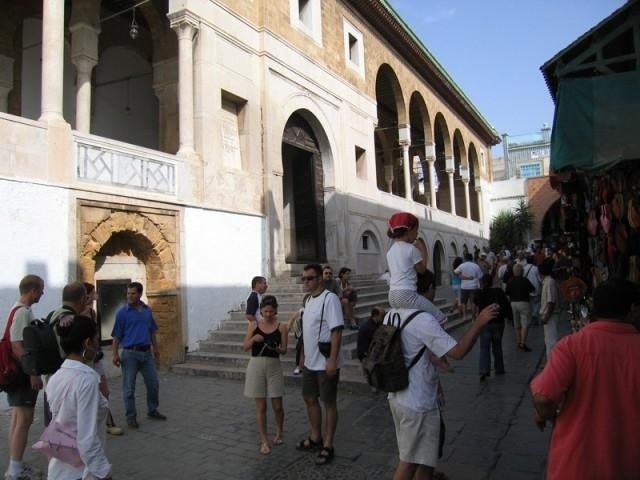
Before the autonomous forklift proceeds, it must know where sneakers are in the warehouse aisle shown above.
[294,367,301,374]
[23,464,44,474]
[4,472,42,480]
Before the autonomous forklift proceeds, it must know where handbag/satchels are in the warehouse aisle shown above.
[32,420,90,467]
[316,340,332,357]
[0,341,26,387]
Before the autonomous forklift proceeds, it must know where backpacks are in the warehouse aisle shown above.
[363,309,429,392]
[22,310,76,375]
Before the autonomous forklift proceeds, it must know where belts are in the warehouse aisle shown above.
[122,344,150,351]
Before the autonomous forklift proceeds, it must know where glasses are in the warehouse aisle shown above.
[301,276,320,281]
[325,272,332,275]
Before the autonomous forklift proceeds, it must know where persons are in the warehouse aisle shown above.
[381,212,462,374]
[245,276,268,324]
[112,278,168,427]
[384,267,503,480]
[43,280,87,436]
[292,293,312,374]
[241,295,288,454]
[1,273,48,480]
[293,263,345,463]
[79,283,124,436]
[381,209,462,374]
[322,265,361,330]
[358,305,385,391]
[44,312,111,480]
[450,240,590,382]
[528,276,639,480]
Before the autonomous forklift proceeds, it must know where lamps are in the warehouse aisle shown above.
[129,8,139,40]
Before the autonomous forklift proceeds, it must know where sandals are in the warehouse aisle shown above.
[260,441,271,455]
[273,435,283,444]
[315,445,334,466]
[295,437,321,450]
[519,343,532,352]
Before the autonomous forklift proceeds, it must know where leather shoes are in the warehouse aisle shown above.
[105,425,123,435]
[127,416,138,427]
[147,409,166,420]
